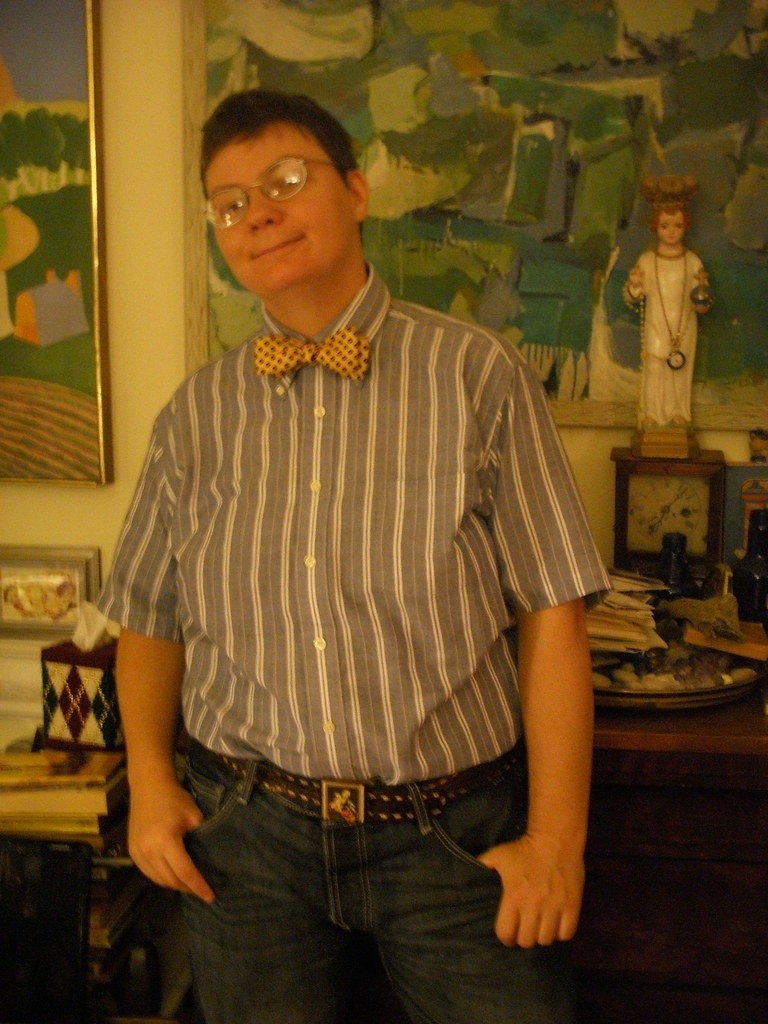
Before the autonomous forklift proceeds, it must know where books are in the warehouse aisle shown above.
[1,737,133,867]
[587,571,672,653]
[683,621,768,660]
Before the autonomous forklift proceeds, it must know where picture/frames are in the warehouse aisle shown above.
[0,0,112,485]
[0,546,99,643]
[180,0,768,429]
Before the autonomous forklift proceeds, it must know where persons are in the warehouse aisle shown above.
[622,175,716,429]
[97,90,614,1024]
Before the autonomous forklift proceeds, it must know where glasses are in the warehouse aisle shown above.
[203,155,335,229]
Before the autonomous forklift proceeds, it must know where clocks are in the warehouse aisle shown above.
[611,446,727,575]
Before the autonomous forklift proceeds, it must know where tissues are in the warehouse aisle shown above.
[40,600,126,752]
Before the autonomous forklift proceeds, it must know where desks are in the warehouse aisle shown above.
[534,706,768,1024]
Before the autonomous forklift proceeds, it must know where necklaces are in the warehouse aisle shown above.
[655,242,688,370]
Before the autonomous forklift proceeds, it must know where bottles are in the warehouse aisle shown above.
[652,532,701,603]
[733,509,768,627]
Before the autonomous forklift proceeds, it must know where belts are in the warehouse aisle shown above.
[195,750,517,825]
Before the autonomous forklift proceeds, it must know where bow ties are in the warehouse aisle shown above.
[254,327,374,384]
